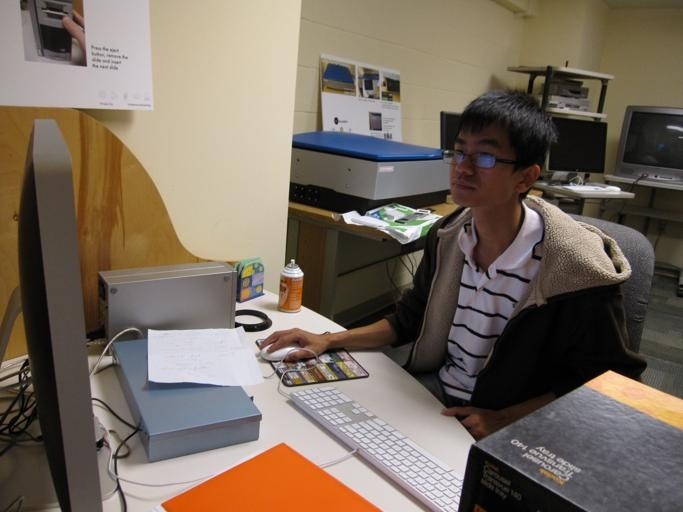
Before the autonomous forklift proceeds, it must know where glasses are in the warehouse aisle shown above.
[439,148,521,170]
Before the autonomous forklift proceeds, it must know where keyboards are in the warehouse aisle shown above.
[289,385,462,512]
[562,185,607,192]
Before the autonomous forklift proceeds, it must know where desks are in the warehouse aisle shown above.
[287,184,542,327]
[2,100,475,512]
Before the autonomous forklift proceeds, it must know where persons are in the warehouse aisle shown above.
[261,88,648,439]
[62,8,86,66]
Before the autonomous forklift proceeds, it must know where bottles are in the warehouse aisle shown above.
[276,255,307,313]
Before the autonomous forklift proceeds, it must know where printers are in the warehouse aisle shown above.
[288,130,451,216]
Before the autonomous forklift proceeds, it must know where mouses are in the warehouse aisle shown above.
[259,341,301,362]
[606,185,621,192]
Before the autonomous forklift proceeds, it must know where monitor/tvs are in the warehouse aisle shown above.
[545,117,608,174]
[616,104,683,186]
[0,119,105,512]
[440,110,463,150]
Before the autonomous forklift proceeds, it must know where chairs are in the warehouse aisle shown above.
[556,209,656,366]
